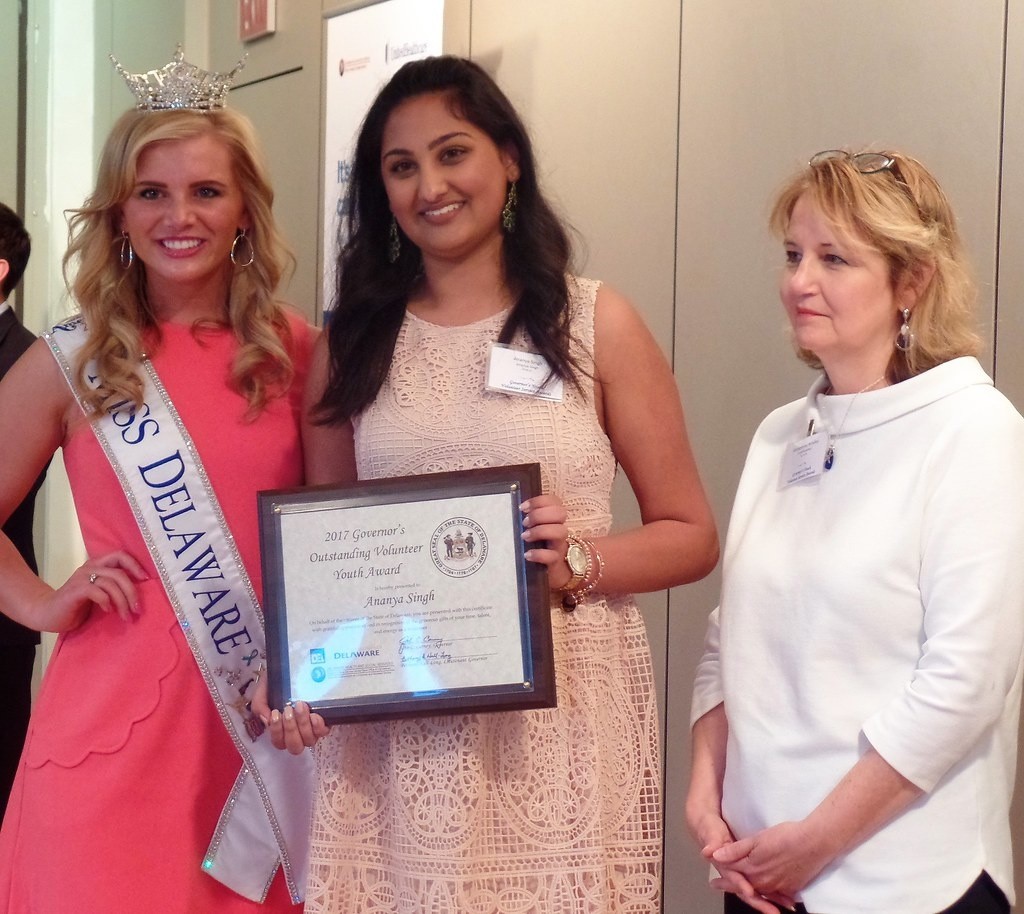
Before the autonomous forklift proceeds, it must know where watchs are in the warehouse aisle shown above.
[554,538,587,594]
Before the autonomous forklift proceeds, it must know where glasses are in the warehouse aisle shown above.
[808,149,927,220]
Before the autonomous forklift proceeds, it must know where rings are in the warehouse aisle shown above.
[88,573,98,583]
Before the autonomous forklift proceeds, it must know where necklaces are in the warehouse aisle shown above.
[813,377,886,469]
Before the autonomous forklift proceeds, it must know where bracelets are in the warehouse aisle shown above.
[561,535,604,613]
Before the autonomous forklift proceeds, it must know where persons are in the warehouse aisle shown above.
[683,153,1024,913]
[251,56,716,913]
[1,203,52,817]
[0,102,308,914]
